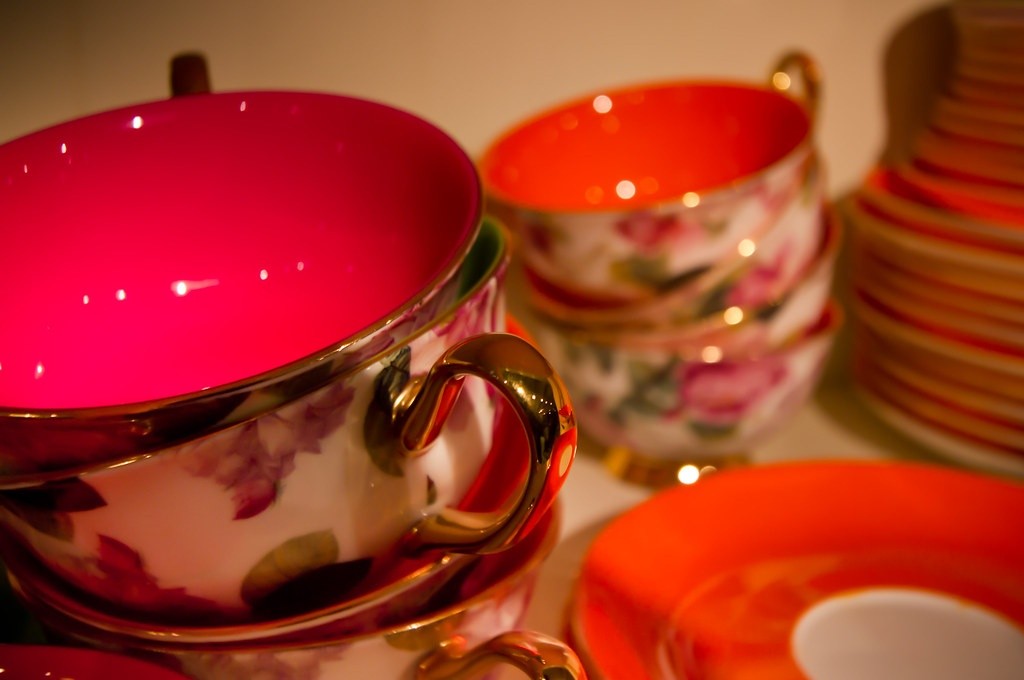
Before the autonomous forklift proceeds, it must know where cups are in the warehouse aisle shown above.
[0,1,847,680]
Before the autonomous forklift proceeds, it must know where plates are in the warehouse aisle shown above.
[556,2,1024,680]
